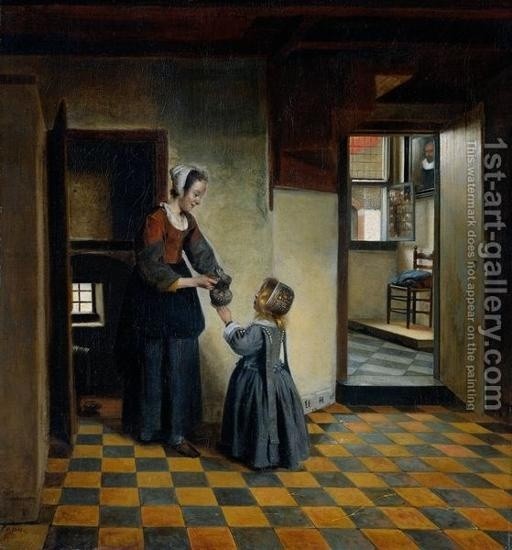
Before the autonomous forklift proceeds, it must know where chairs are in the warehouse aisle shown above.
[386,243,435,329]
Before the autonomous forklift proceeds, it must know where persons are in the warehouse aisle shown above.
[112,163,232,458]
[216,278,313,469]
[415,140,435,193]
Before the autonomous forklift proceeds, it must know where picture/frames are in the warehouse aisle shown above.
[403,135,435,200]
